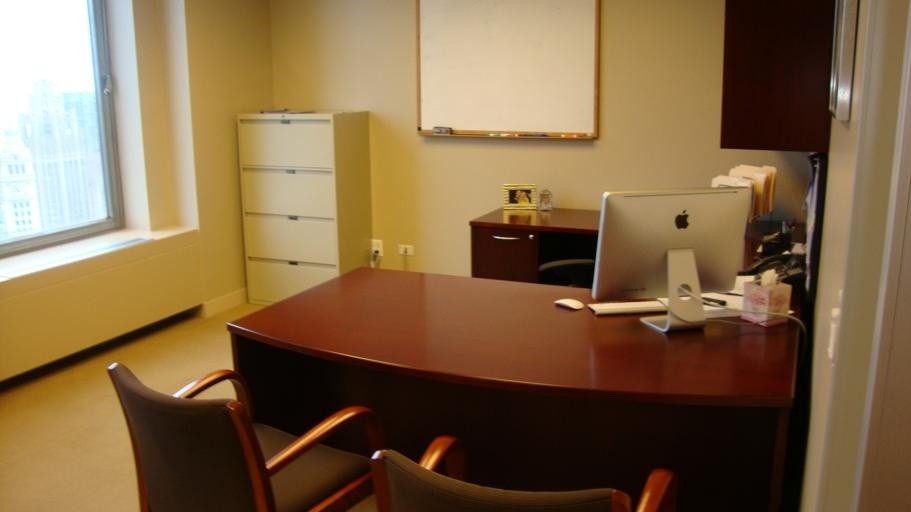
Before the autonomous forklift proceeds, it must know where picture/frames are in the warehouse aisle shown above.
[503,183,537,209]
[538,189,553,210]
[829,0,857,122]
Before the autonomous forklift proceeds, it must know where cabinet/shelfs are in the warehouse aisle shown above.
[238,111,372,306]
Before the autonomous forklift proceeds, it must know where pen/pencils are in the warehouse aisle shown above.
[701,297,728,306]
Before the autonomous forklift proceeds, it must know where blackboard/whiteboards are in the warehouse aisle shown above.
[415,0,600,139]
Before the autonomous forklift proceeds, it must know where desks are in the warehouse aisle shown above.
[469,206,807,282]
[226,268,797,509]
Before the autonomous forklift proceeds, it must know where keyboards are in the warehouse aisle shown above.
[587,300,668,317]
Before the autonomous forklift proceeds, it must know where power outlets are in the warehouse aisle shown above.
[372,239,383,256]
[399,245,414,255]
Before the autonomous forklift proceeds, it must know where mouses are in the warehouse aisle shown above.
[553,298,584,310]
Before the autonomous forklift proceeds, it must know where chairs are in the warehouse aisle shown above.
[370,435,675,512]
[106,362,380,512]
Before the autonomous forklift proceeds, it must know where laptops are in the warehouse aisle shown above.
[591,187,752,334]
